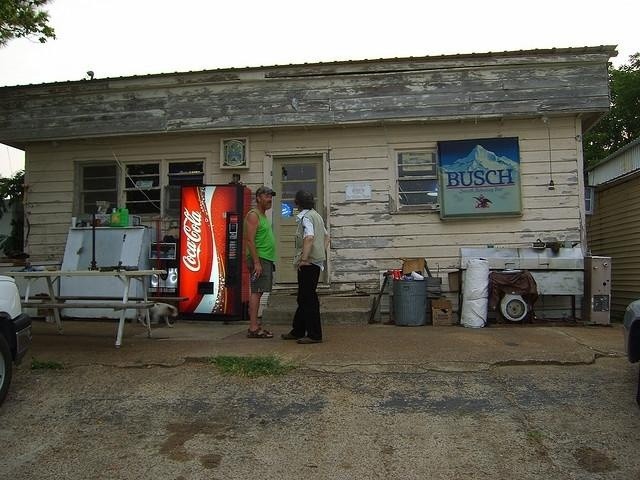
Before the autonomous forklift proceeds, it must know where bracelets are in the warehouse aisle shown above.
[301,258,308,262]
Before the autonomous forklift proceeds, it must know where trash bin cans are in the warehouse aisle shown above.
[393,279,427,326]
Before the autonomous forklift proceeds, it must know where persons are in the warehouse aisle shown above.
[243,185,277,339]
[281,190,331,344]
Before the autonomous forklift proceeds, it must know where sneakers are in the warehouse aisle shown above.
[281,332,322,344]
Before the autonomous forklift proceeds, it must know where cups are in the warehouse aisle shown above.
[72,217,77,227]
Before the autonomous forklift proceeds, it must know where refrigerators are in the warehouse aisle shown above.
[179,183,251,321]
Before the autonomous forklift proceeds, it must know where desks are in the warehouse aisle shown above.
[24,269,167,349]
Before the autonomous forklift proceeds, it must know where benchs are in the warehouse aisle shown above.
[21,296,189,349]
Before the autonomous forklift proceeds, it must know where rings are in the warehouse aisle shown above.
[254,273,256,275]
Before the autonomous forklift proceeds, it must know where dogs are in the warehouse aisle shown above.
[136,301,178,328]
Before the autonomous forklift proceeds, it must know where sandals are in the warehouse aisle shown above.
[247,327,273,338]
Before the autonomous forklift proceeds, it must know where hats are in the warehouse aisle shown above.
[256,186,276,197]
[296,190,316,204]
